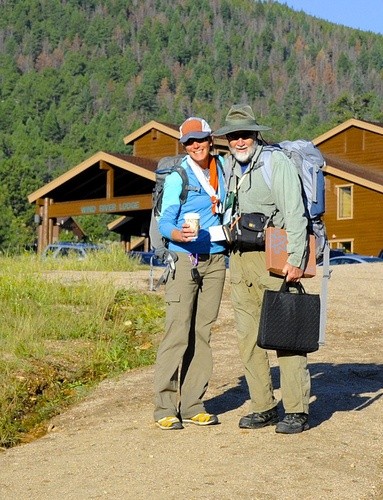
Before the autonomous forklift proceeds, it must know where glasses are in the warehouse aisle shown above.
[183,136,207,146]
[229,131,253,140]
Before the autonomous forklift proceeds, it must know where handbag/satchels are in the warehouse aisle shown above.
[257,276,320,353]
[231,211,274,247]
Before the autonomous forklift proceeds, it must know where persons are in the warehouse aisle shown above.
[211,103,311,434]
[154,117,229,429]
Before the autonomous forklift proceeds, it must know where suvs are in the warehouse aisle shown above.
[42,241,166,267]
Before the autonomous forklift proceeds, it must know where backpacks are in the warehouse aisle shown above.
[150,154,224,257]
[228,140,325,259]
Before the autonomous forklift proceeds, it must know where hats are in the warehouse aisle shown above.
[210,105,273,136]
[179,117,212,142]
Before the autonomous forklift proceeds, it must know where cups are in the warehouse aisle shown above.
[184,213,200,241]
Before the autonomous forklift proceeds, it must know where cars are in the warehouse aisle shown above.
[316,249,383,266]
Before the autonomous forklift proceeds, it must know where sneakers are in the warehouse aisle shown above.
[239,406,281,428]
[155,416,184,429]
[275,413,310,434]
[182,411,219,425]
